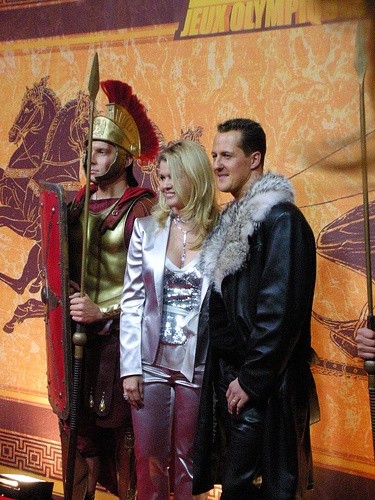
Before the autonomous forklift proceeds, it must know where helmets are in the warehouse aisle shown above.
[82,80,159,185]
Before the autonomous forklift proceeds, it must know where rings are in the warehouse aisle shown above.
[232,399,238,403]
[123,393,128,399]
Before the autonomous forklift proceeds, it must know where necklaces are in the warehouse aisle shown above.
[172,215,193,264]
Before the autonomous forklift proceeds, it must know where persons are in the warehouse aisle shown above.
[39,78,161,500]
[193,118,317,500]
[119,140,228,500]
